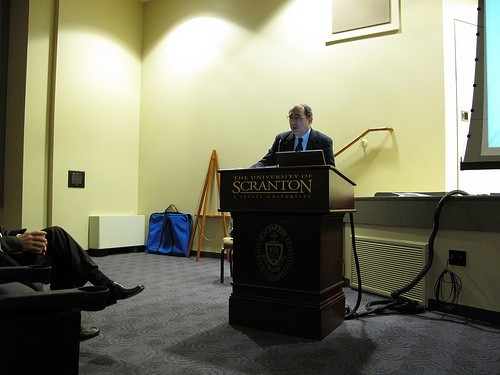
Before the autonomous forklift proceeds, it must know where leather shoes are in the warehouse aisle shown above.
[107,282,145,307]
[79,326,101,340]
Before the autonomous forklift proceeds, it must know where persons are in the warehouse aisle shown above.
[249,103,336,169]
[0,226,145,341]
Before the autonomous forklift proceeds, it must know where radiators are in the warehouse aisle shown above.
[349,233,431,309]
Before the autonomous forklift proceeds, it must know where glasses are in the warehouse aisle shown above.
[288,116,306,121]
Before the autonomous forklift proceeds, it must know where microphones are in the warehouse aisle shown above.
[277,135,283,166]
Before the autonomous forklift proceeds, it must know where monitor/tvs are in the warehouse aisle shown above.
[276,150,326,166]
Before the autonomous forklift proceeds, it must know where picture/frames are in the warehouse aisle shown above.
[325,0,401,46]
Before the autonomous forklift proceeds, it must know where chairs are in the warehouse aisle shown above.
[220,220,233,284]
[0,264,109,375]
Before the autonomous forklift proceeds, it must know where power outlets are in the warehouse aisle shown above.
[448,250,467,267]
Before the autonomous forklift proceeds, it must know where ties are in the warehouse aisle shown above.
[294,138,304,152]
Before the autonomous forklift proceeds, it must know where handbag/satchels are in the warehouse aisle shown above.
[146,204,192,257]
